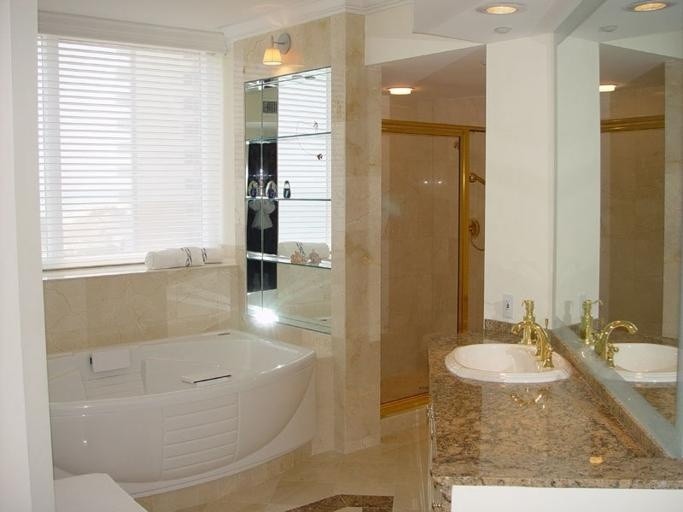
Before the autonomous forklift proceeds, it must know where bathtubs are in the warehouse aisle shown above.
[46,328,318,501]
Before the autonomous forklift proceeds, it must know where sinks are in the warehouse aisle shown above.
[446,342,572,384]
[606,342,680,386]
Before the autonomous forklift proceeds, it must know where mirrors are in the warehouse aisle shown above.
[550,0,682,467]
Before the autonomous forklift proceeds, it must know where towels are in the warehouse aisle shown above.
[201,247,223,264]
[278,241,329,259]
[145,248,201,270]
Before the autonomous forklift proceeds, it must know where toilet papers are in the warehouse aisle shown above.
[89,347,131,373]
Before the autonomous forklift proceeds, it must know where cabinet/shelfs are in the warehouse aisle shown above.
[246,67,335,332]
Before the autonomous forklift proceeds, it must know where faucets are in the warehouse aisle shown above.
[510,320,551,357]
[595,317,639,355]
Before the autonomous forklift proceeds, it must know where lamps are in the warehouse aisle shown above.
[262,32,290,66]
